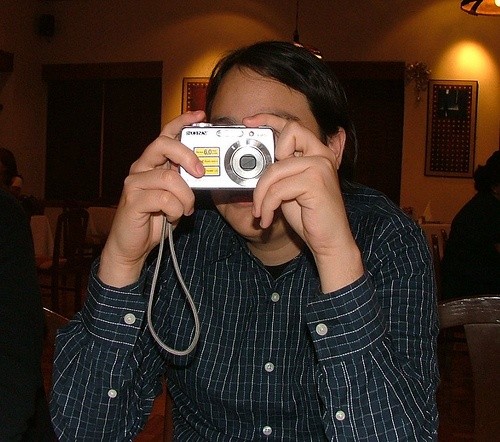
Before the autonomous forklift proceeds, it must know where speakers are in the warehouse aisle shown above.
[39,15,55,37]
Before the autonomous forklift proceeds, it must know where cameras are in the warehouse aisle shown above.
[178,121,276,190]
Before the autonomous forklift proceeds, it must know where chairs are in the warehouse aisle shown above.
[36,196,103,317]
[420,223,500,442]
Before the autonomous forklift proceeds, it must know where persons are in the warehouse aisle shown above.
[49,41,441,442]
[0,148,55,442]
[440,151,500,300]
